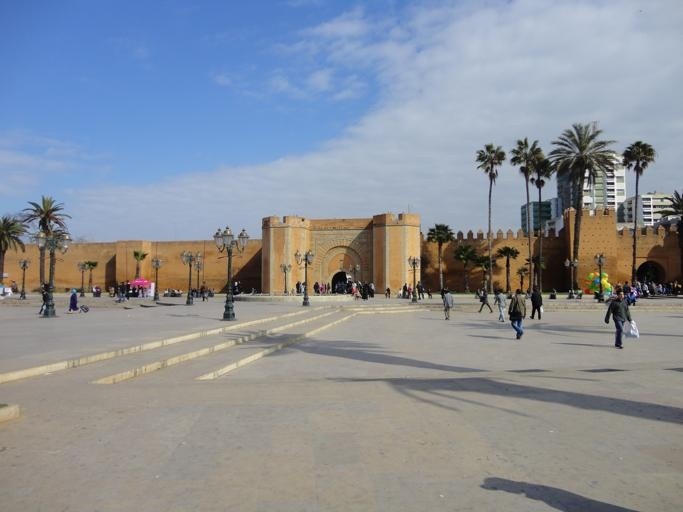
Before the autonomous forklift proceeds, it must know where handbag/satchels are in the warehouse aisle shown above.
[511,304,521,317]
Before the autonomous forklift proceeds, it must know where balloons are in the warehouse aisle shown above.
[584,271,611,295]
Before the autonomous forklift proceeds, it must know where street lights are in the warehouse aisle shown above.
[20,259,30,299]
[181,249,200,304]
[349,264,360,283]
[37,233,72,316]
[280,264,292,292]
[408,257,420,302]
[152,259,160,300]
[213,225,250,321]
[295,249,314,305]
[594,253,606,302]
[565,258,577,298]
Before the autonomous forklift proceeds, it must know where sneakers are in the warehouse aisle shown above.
[530,316,533,319]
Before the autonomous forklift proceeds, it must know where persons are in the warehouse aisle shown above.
[604,289,632,349]
[507,289,526,339]
[108,280,146,299]
[442,287,452,320]
[199,280,208,302]
[10,280,18,293]
[493,287,506,322]
[68,288,79,311]
[529,286,542,320]
[294,280,431,300]
[477,287,492,314]
[548,280,682,307]
